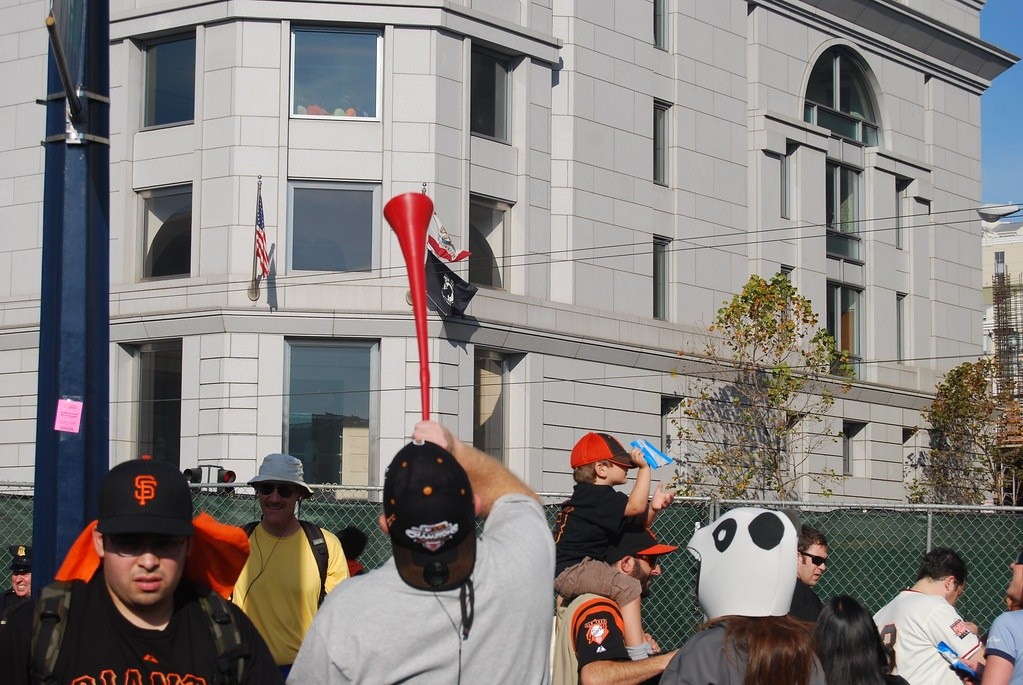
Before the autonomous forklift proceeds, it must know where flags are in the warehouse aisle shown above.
[254,188,270,279]
[426,209,472,262]
[425,250,479,318]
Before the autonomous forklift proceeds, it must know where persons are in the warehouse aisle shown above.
[552,521,681,685]
[227,453,350,680]
[0,454,286,685]
[872,548,1023,685]
[814,594,910,685]
[658,507,828,685]
[551,432,677,661]
[0,546,34,631]
[336,526,367,576]
[284,419,557,685]
[789,524,828,622]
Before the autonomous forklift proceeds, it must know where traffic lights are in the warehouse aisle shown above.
[184,467,202,492]
[217,469,236,496]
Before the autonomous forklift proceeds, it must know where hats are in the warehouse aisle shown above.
[97,455,194,537]
[8,545,32,571]
[384,441,476,590]
[604,524,678,564]
[571,432,638,468]
[247,454,314,499]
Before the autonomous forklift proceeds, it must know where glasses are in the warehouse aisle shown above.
[801,552,828,567]
[1015,550,1023,564]
[633,554,657,569]
[105,535,186,557]
[258,484,292,498]
